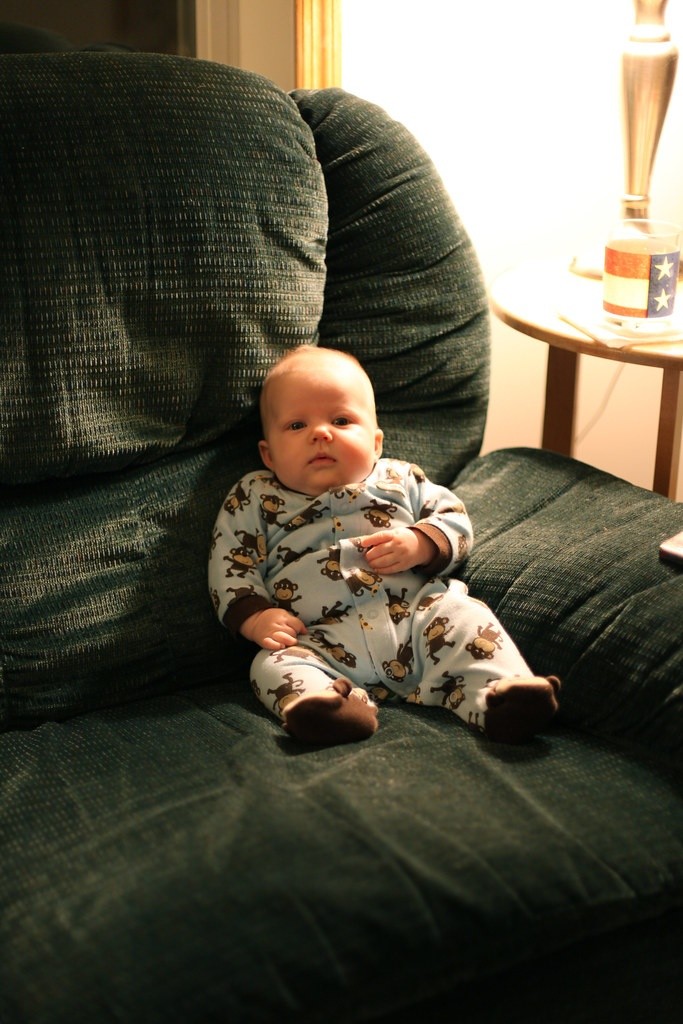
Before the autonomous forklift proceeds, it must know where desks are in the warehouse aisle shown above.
[492,274,683,502]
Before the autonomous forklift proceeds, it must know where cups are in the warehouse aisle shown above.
[601,219,683,338]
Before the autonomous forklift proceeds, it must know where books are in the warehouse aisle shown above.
[558,295,683,349]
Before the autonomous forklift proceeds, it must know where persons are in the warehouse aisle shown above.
[208,346,559,747]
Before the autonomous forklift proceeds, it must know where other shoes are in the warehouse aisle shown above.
[283,679,376,740]
[485,676,561,743]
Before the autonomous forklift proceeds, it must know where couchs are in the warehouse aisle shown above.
[0,48,683,1024]
